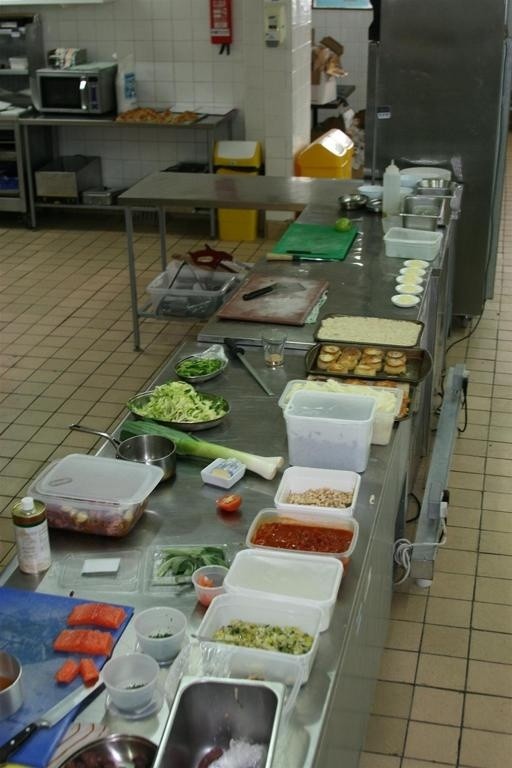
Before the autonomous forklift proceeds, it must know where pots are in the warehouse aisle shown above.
[69,423,177,482]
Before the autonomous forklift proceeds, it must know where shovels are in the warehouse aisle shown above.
[243,283,305,300]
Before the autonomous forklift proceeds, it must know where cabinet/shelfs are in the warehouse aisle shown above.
[1,11,47,214]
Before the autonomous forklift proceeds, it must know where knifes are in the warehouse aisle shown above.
[0,671,103,761]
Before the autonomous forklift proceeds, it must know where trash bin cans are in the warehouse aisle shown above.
[297,127,354,178]
[213,140,261,241]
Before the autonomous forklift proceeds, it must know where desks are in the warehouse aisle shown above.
[26,107,237,240]
[312,84,356,132]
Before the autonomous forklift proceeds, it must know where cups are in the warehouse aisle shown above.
[0,649,23,722]
[192,564,231,604]
[102,653,160,710]
[135,608,187,657]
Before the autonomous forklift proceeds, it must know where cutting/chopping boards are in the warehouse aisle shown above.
[217,270,329,327]
[273,222,358,261]
[1,586,137,768]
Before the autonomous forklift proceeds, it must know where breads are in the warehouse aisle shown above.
[317,345,406,376]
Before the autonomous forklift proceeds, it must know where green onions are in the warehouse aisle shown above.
[122,420,285,480]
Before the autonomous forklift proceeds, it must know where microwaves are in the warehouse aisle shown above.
[35,66,116,114]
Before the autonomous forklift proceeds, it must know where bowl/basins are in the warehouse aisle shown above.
[391,255,430,307]
[400,166,458,197]
[338,182,383,210]
[61,732,161,768]
[128,390,230,430]
[174,351,230,381]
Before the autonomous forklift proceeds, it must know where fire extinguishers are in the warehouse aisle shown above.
[210,0,233,55]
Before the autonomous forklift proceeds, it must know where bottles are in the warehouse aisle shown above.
[382,157,400,217]
[12,496,50,574]
[261,331,287,365]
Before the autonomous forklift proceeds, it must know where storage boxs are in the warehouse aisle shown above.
[147,271,239,319]
